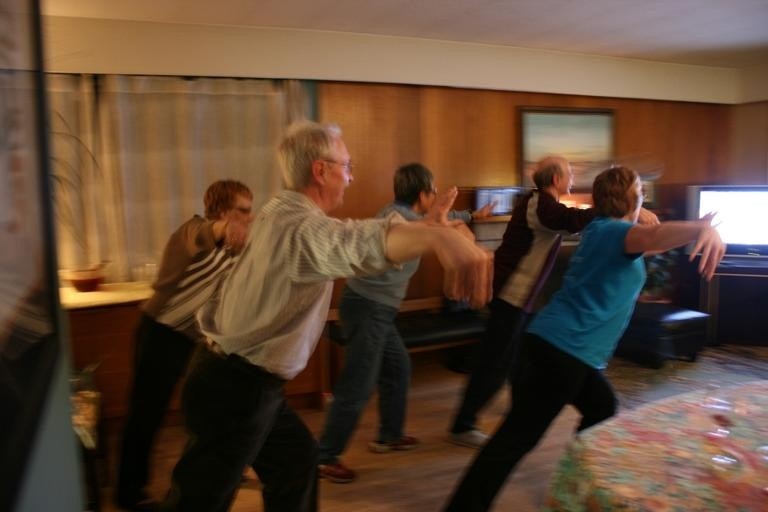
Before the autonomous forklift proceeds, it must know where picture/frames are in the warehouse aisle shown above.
[517,104,619,196]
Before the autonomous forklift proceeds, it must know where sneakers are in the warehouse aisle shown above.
[367,435,420,454]
[444,430,487,450]
[317,461,356,483]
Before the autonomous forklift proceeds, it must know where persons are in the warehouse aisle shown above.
[443,165,727,512]
[163,123,494,512]
[113,179,255,512]
[317,162,498,484]
[443,155,660,452]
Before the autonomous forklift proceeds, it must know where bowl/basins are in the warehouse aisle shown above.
[67,270,109,290]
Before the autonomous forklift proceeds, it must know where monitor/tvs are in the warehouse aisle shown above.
[685,185,767,268]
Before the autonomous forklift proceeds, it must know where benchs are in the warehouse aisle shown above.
[327,294,491,425]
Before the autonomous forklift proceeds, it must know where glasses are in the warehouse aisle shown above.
[322,157,355,172]
[235,206,252,213]
[430,187,438,194]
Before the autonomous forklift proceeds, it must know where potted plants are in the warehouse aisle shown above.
[638,249,680,318]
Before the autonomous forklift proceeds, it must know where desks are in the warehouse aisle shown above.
[698,258,768,343]
[537,377,768,512]
[59,281,155,418]
